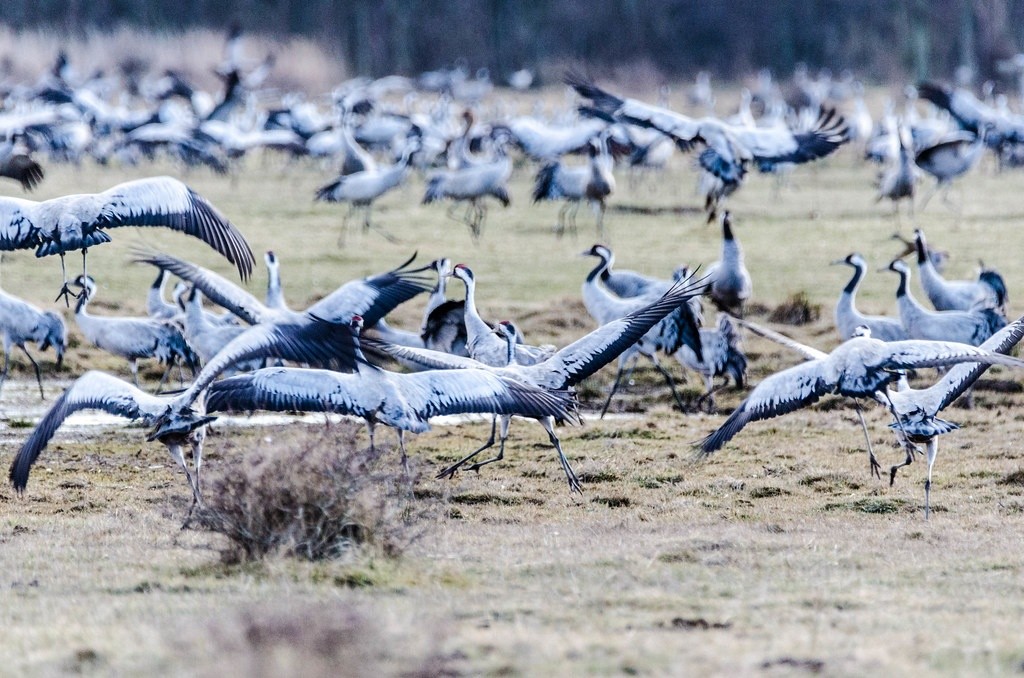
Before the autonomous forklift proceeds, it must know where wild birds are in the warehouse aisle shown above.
[0,21,1023,529]
